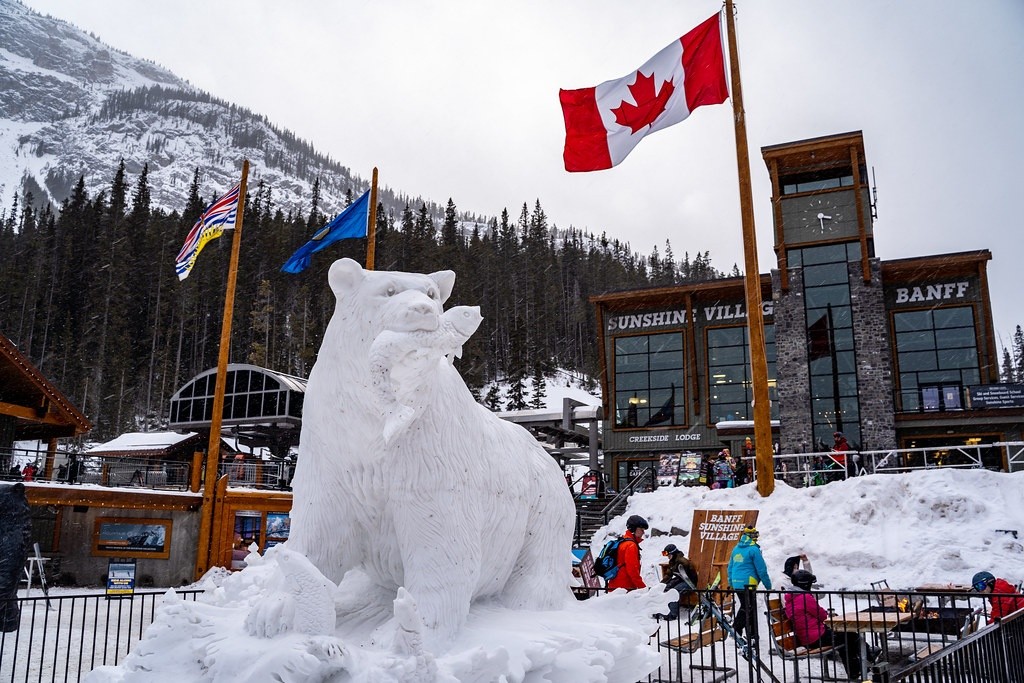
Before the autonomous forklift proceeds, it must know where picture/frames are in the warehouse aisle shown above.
[91,517,173,559]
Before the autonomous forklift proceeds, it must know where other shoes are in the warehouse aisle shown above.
[867,646,883,663]
[656,613,676,620]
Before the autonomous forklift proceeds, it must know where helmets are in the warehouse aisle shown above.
[664,543,679,554]
[834,432,843,437]
[791,570,817,590]
[972,571,996,589]
[626,516,648,532]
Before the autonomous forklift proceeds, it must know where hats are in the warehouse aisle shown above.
[744,525,759,542]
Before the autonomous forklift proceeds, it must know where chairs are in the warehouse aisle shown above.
[764,589,850,683]
[870,579,901,608]
[653,600,736,683]
[908,608,982,663]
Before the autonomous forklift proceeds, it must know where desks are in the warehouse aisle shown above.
[822,612,916,681]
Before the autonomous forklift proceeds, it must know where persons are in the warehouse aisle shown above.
[971,571,1024,624]
[830,432,850,481]
[700,449,747,490]
[0,483,31,633]
[131,470,145,486]
[10,464,79,485]
[658,545,698,621]
[608,516,648,595]
[775,554,881,683]
[727,526,771,651]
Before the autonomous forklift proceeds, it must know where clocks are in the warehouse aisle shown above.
[802,200,843,232]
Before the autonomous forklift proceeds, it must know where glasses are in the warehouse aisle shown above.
[662,551,668,556]
[973,578,987,591]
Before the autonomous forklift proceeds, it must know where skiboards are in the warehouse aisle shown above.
[683,569,721,626]
[673,563,781,683]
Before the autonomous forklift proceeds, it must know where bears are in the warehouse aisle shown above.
[244,257,576,653]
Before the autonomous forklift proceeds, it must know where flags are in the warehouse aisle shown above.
[176,183,241,283]
[559,6,732,173]
[281,189,371,274]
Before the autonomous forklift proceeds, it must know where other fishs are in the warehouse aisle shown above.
[274,540,339,637]
[372,305,485,366]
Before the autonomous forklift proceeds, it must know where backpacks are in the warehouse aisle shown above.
[594,538,639,580]
